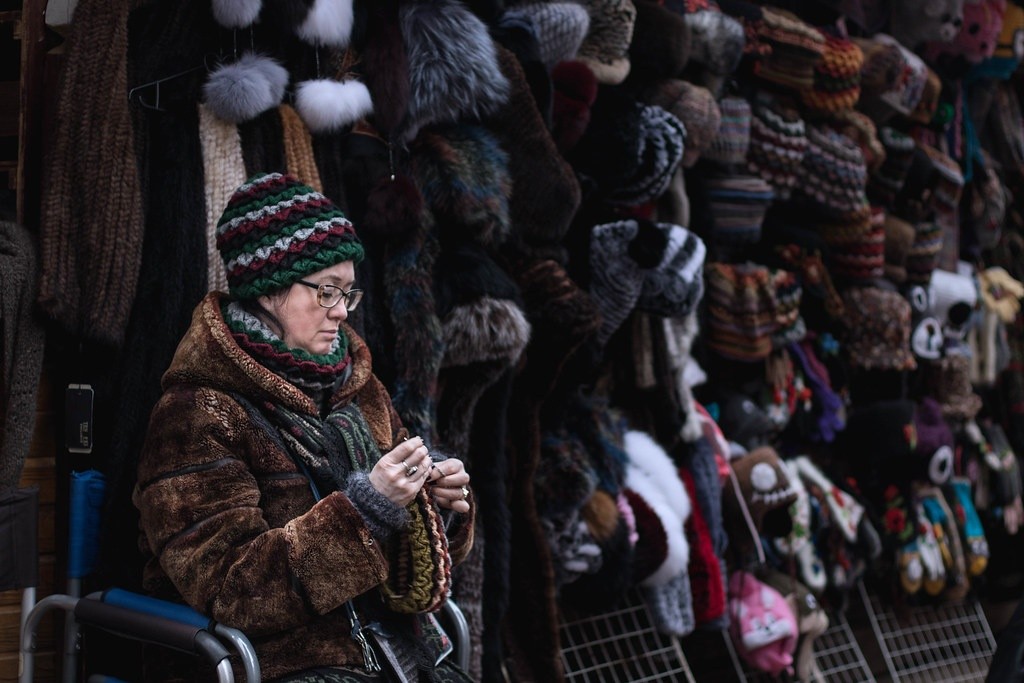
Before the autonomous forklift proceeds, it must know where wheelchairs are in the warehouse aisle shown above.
[14,311,470,683]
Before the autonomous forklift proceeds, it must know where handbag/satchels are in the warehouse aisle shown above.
[364,616,423,683]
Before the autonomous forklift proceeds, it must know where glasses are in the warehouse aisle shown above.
[296,279,364,313]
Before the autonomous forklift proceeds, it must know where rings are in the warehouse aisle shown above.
[461,488,469,499]
[402,462,418,477]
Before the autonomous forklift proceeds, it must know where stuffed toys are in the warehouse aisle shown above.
[890,1,1024,79]
[726,264,1024,629]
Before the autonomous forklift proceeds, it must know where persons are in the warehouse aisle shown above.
[133,176,475,683]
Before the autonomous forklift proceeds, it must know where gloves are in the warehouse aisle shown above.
[791,342,846,445]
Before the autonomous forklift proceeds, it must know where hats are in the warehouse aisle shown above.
[363,1,1024,682]
[215,172,366,304]
